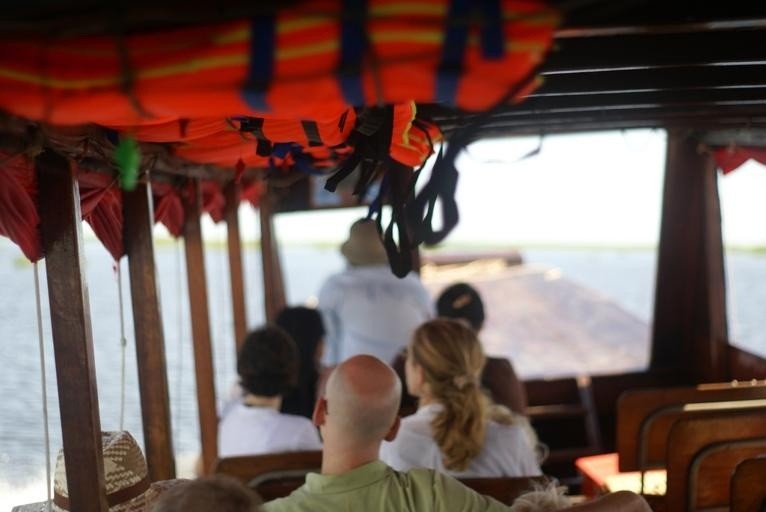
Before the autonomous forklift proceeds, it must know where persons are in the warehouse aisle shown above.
[52,214,653,512]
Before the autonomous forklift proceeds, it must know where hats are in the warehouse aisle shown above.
[12,430,193,512]
[341,218,390,264]
